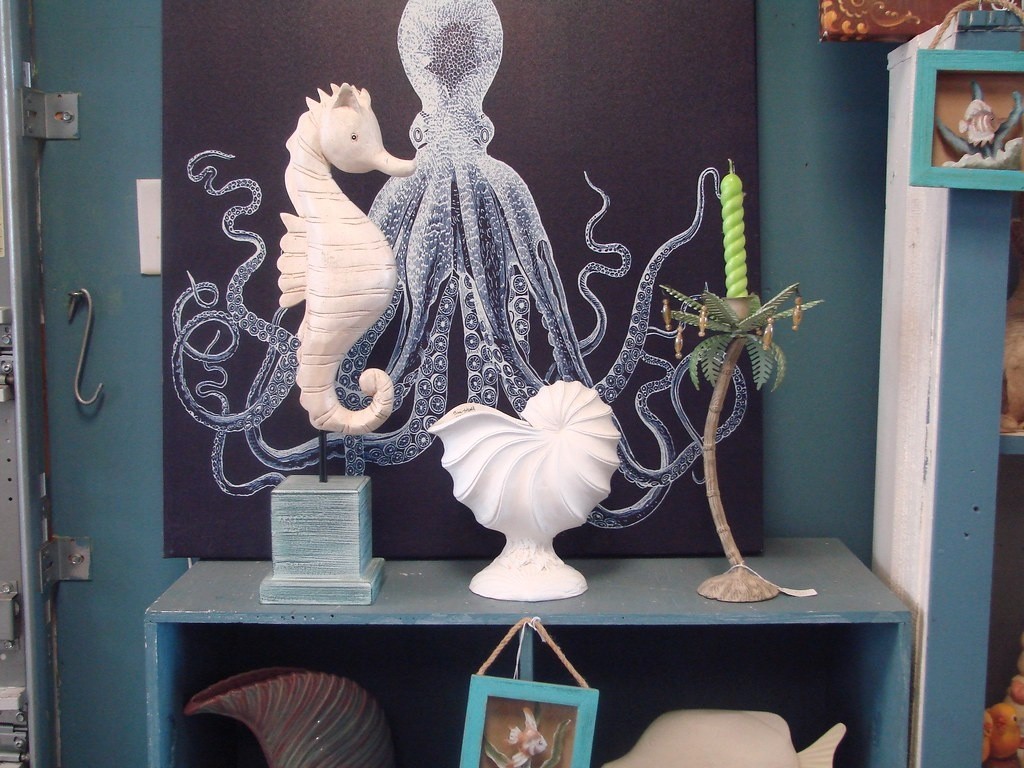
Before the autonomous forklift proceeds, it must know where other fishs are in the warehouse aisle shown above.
[505,707,549,767]
[958,99,1012,148]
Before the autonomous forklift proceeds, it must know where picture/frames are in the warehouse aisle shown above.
[908,49,1024,192]
[459,674,599,768]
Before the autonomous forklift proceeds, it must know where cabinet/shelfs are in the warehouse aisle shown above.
[144,537,911,767]
[871,7,1024,768]
